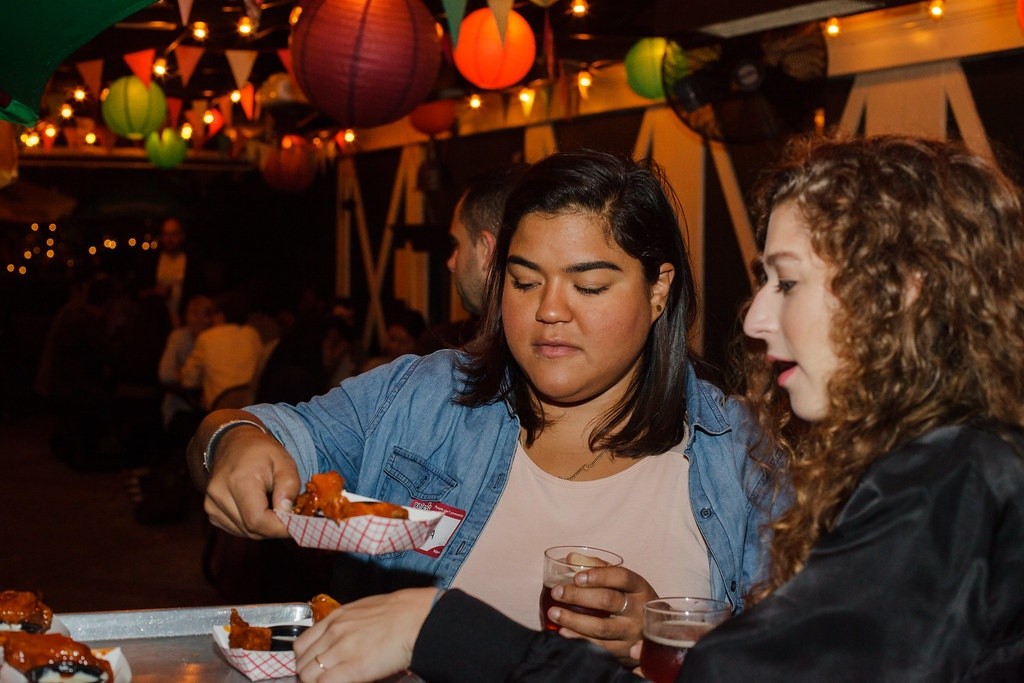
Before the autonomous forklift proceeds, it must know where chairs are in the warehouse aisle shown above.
[210,384,250,412]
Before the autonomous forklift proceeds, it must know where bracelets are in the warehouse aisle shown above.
[203,419,268,475]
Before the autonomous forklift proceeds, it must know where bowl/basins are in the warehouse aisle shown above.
[266,625,312,651]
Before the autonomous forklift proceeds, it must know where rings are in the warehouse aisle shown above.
[315,656,327,672]
[617,593,633,616]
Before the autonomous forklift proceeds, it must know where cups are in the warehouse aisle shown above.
[539,546,623,634]
[639,597,733,683]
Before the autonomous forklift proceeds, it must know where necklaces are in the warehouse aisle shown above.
[517,430,611,481]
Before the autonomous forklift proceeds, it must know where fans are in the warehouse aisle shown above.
[658,0,829,147]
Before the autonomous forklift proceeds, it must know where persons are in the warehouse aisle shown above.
[54,218,425,491]
[183,149,797,660]
[293,133,1024,683]
